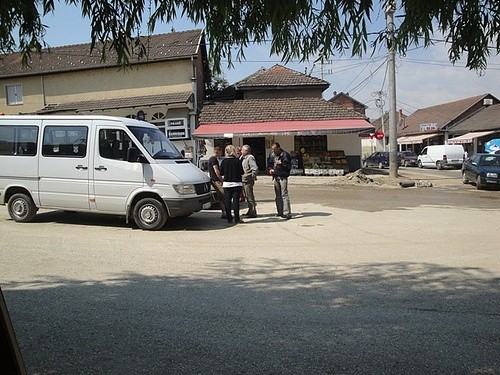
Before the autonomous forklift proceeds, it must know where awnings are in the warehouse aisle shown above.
[396,133,440,144]
[191,120,376,142]
[447,131,496,144]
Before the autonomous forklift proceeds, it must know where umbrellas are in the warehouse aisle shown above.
[484,138,500,153]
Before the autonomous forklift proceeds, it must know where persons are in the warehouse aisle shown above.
[268,142,292,219]
[127,142,144,161]
[478,142,485,152]
[296,148,306,176]
[219,145,245,224]
[236,147,246,203]
[241,145,258,218]
[209,146,234,219]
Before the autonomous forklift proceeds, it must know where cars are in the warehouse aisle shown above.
[397,150,419,167]
[460,152,500,190]
[362,151,402,169]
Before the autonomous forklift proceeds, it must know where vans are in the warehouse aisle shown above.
[0,114,212,231]
[417,145,465,170]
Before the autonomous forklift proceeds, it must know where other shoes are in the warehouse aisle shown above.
[221,215,233,218]
[235,219,244,223]
[228,220,233,223]
[282,214,292,219]
[243,213,257,218]
[276,214,283,217]
